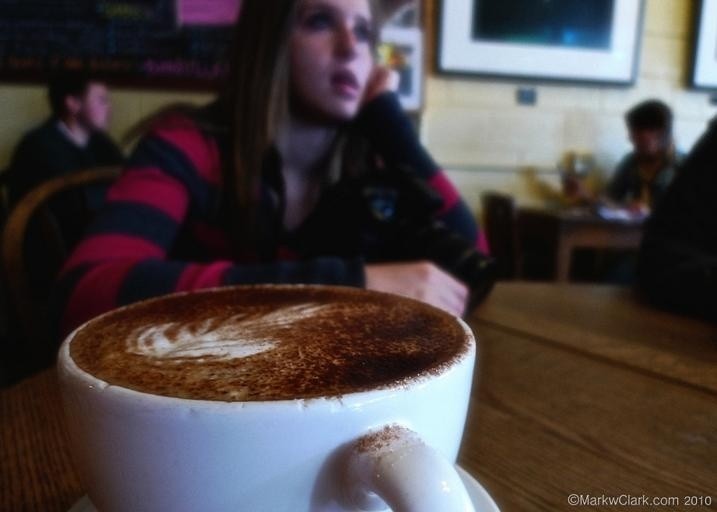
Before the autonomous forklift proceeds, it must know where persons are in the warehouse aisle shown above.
[641,116,717,318]
[48,0,500,317]
[8,69,122,192]
[601,98,687,220]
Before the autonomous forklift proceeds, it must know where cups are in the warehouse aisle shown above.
[57,284,505,512]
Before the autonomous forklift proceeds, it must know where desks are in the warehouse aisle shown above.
[0,189,716,510]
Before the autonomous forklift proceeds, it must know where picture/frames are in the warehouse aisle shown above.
[434,0,644,88]
[686,1,717,95]
